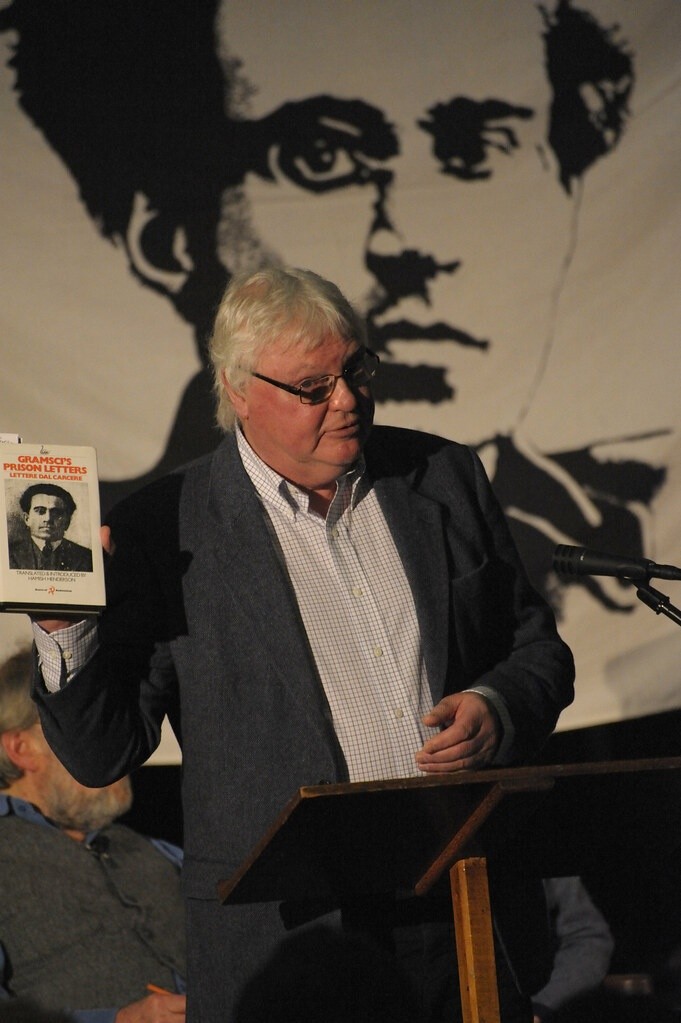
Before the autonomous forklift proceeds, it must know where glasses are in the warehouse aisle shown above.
[242,346,381,404]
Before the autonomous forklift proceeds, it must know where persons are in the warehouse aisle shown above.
[24,265,577,1023]
[0,638,184,1023]
[9,484,92,573]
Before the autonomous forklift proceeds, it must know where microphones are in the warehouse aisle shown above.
[553,544,681,580]
